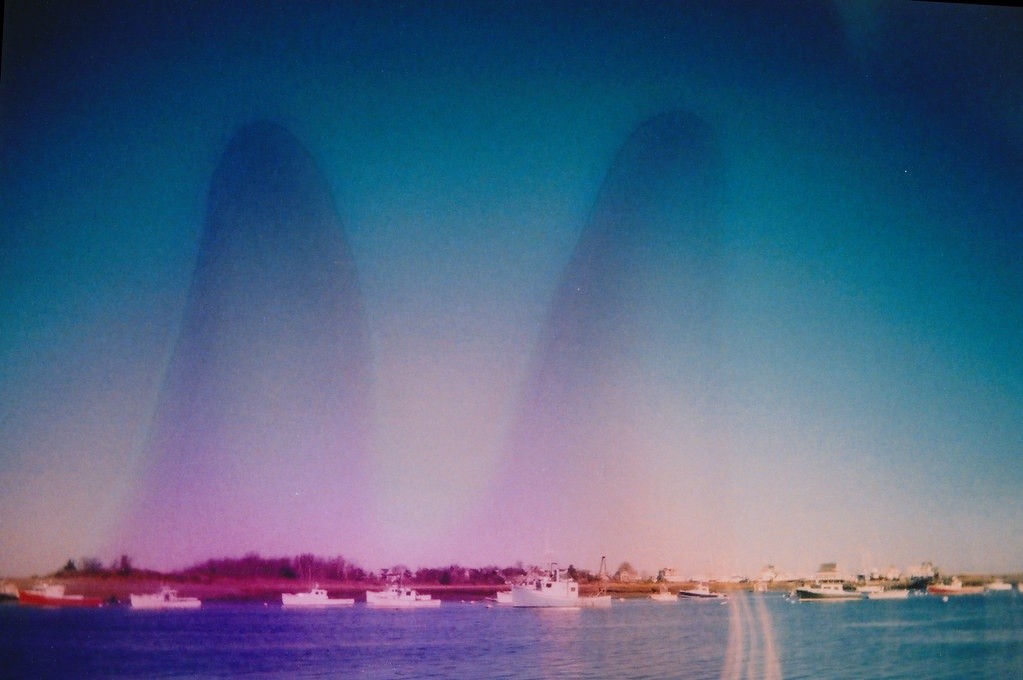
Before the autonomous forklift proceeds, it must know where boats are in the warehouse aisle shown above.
[281,585,354,605]
[496,590,512,604]
[366,576,442,608]
[129,584,202,609]
[926,583,985,595]
[16,582,103,608]
[795,580,863,598]
[680,583,726,597]
[509,561,612,607]
[982,578,1013,590]
[650,583,678,601]
[867,588,910,599]
